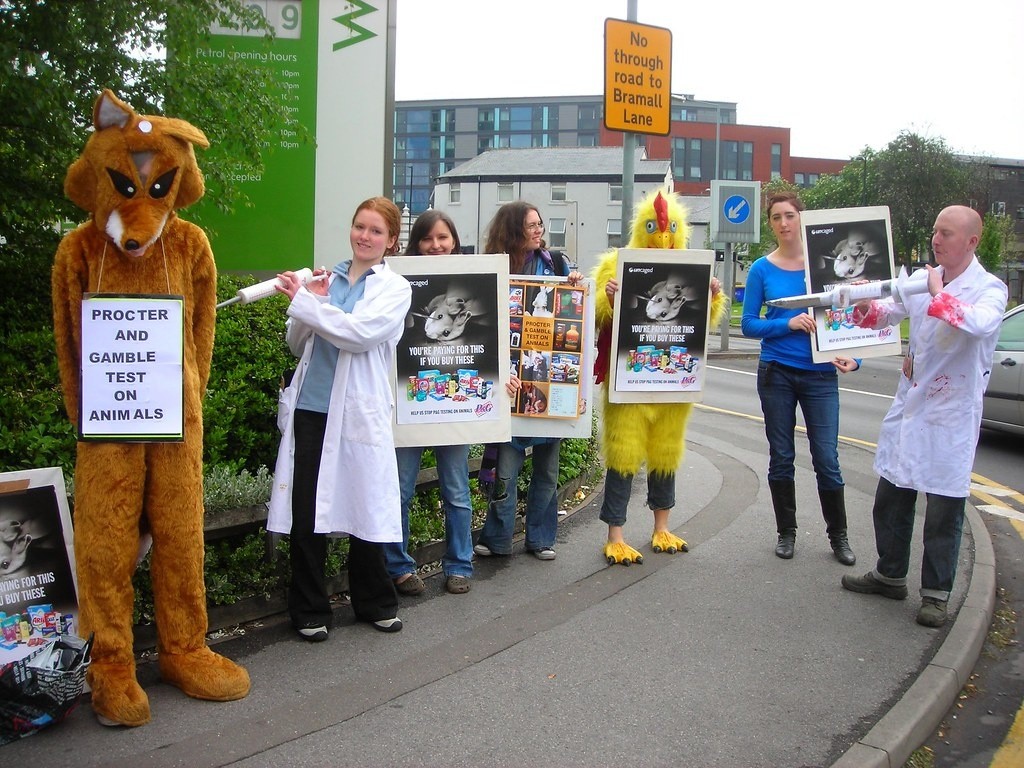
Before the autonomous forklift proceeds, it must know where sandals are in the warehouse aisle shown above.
[395,573,424,593]
[446,575,470,593]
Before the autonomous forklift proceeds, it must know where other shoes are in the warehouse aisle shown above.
[295,618,327,641]
[474,544,491,555]
[370,608,403,632]
[534,548,556,559]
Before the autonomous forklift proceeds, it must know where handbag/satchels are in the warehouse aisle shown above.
[0,621,95,745]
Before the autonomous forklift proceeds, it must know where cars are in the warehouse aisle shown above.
[980,302,1023,434]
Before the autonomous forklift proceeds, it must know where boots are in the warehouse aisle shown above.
[768,479,798,558]
[818,486,856,564]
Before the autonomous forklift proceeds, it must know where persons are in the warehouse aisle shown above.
[840,203,1009,628]
[740,190,860,567]
[475,201,587,560]
[287,196,413,640]
[391,210,476,595]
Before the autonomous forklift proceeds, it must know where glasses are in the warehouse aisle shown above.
[525,223,544,232]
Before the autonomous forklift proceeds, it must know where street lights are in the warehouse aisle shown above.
[565,200,578,274]
[856,155,866,205]
[671,94,722,261]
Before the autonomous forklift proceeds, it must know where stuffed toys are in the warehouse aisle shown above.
[52,87,251,728]
[595,187,727,568]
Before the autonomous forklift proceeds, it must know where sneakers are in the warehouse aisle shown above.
[842,572,908,600]
[917,596,948,626]
